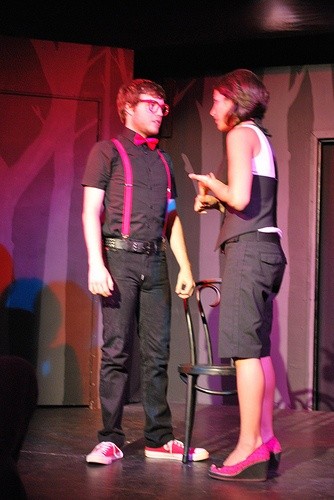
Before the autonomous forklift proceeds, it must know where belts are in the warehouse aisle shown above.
[227,235,239,243]
[105,237,162,255]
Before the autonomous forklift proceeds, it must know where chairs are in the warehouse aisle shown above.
[178,278,237,464]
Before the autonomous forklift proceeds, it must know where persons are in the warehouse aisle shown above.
[82,79,210,464]
[189,69,288,482]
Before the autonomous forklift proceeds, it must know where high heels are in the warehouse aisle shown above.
[207,443,270,481]
[265,435,283,478]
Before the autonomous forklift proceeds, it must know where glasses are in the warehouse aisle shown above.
[139,100,169,116]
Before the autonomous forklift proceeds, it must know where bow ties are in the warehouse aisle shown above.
[134,132,159,151]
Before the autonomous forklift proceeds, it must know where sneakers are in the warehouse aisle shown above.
[85,442,123,464]
[145,438,209,461]
